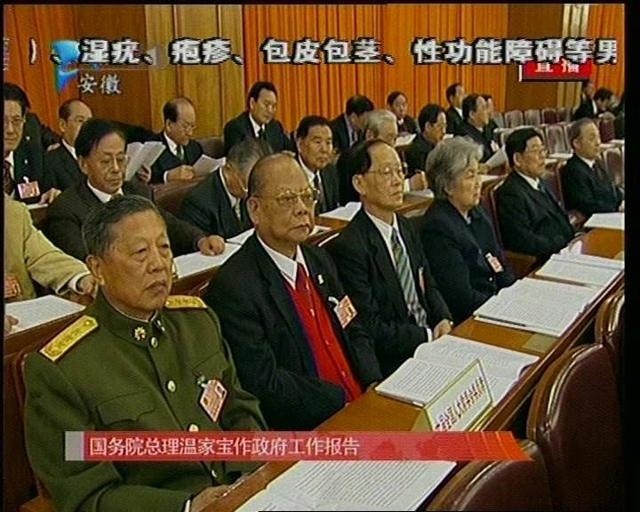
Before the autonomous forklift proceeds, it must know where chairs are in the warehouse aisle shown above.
[0,105,624,512]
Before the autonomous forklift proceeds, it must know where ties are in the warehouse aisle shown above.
[174,145,183,160]
[258,126,267,141]
[391,228,427,329]
[295,263,313,310]
[3,160,16,196]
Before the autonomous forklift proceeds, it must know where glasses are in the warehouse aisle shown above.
[363,163,408,179]
[3,115,26,126]
[255,187,319,209]
[82,155,131,168]
